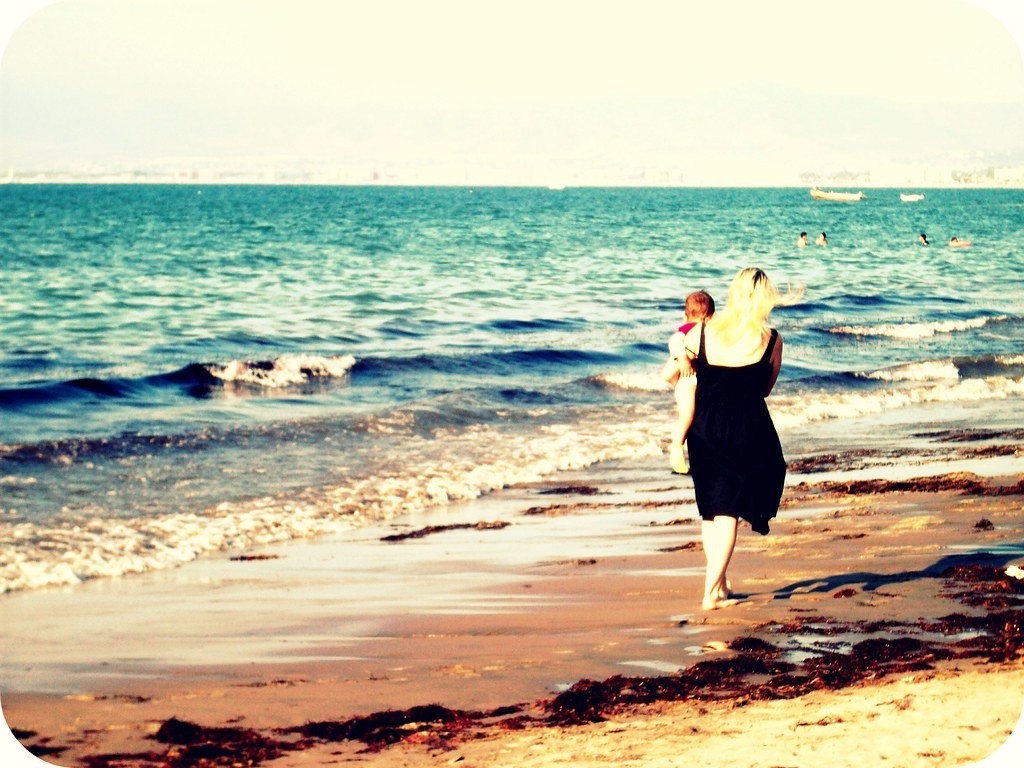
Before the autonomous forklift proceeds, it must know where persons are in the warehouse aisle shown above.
[683,268,783,611]
[919,234,929,246]
[816,232,828,245]
[669,292,714,474]
[797,232,807,247]
[949,236,969,247]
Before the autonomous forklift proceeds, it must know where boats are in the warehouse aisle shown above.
[900,191,928,202]
[809,184,866,205]
